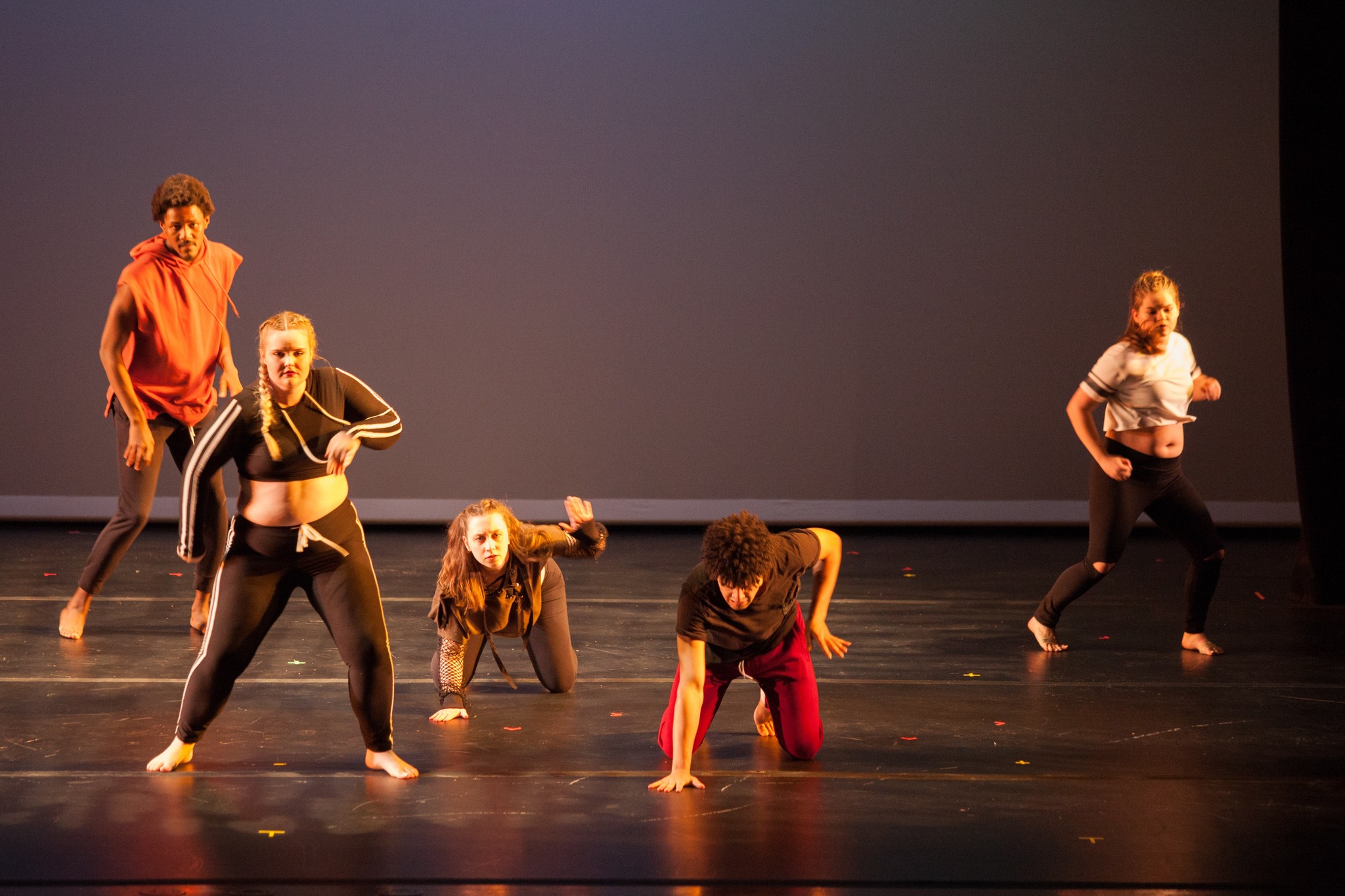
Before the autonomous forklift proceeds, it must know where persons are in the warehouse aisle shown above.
[150,309,418,781]
[1028,271,1223,656]
[57,176,244,638]
[425,497,609,723]
[648,510,851,793]
